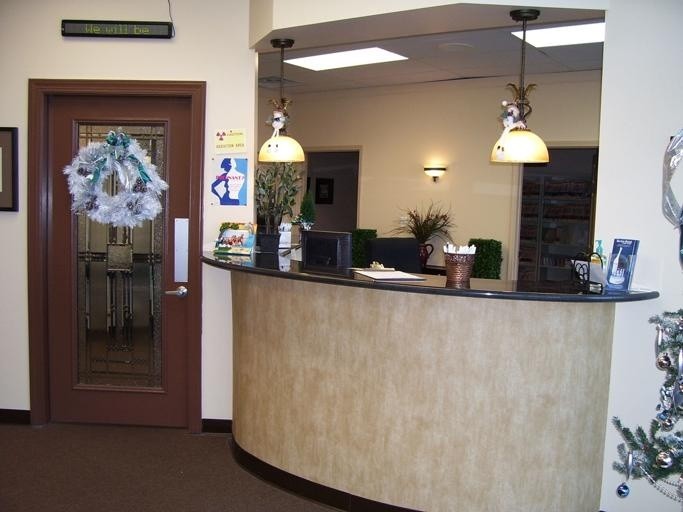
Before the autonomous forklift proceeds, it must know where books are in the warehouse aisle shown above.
[605,238,639,289]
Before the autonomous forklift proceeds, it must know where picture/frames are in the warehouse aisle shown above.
[315,177,334,205]
[0,127,19,212]
[214,222,258,255]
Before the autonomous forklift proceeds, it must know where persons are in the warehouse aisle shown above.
[221,234,244,245]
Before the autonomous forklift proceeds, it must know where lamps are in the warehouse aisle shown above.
[423,160,447,181]
[258,38,306,163]
[490,9,550,164]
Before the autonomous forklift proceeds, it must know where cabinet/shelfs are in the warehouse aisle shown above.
[518,176,543,281]
[537,175,594,281]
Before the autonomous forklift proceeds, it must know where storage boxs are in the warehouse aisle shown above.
[541,265,572,282]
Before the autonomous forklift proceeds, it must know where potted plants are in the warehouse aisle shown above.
[382,198,458,268]
[254,162,306,254]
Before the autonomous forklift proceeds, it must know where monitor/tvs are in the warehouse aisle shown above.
[301,230,351,276]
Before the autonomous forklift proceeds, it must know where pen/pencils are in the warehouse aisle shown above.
[443,242,478,254]
[278,222,293,231]
[297,222,315,231]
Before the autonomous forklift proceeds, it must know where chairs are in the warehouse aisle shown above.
[369,239,421,273]
